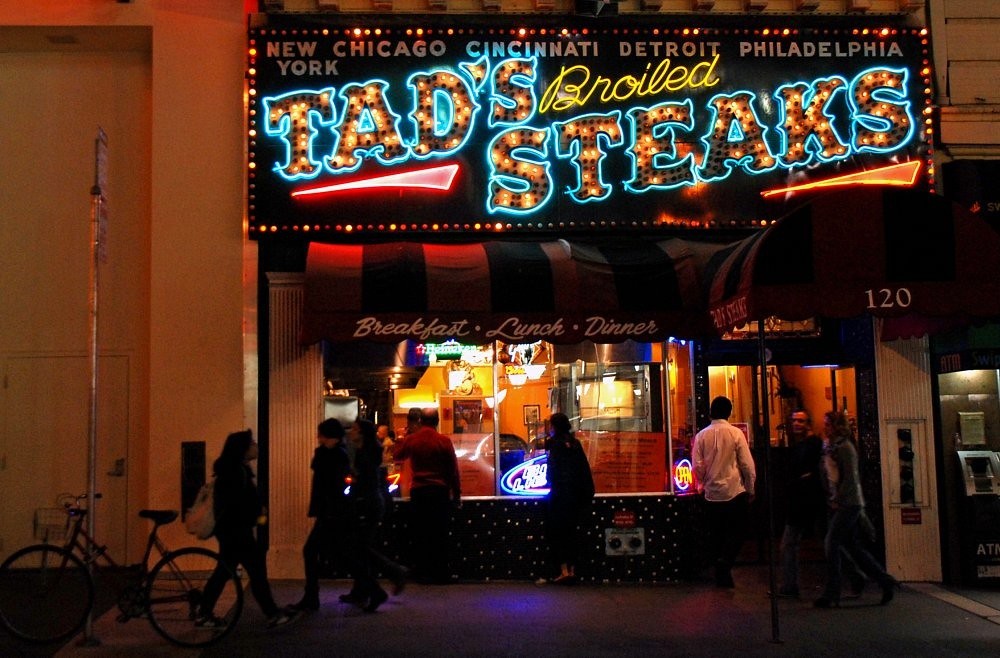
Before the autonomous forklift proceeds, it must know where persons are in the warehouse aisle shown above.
[338,416,412,602]
[285,416,388,615]
[691,394,759,589]
[192,428,294,629]
[393,406,464,586]
[811,409,898,610]
[374,424,395,469]
[544,410,598,585]
[766,410,866,599]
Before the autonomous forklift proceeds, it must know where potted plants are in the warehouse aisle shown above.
[773,376,803,411]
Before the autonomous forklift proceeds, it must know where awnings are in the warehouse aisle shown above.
[295,185,974,353]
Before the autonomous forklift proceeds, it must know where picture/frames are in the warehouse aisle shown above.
[523,404,541,426]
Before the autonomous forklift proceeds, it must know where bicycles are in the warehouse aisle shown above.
[1,494,244,648]
